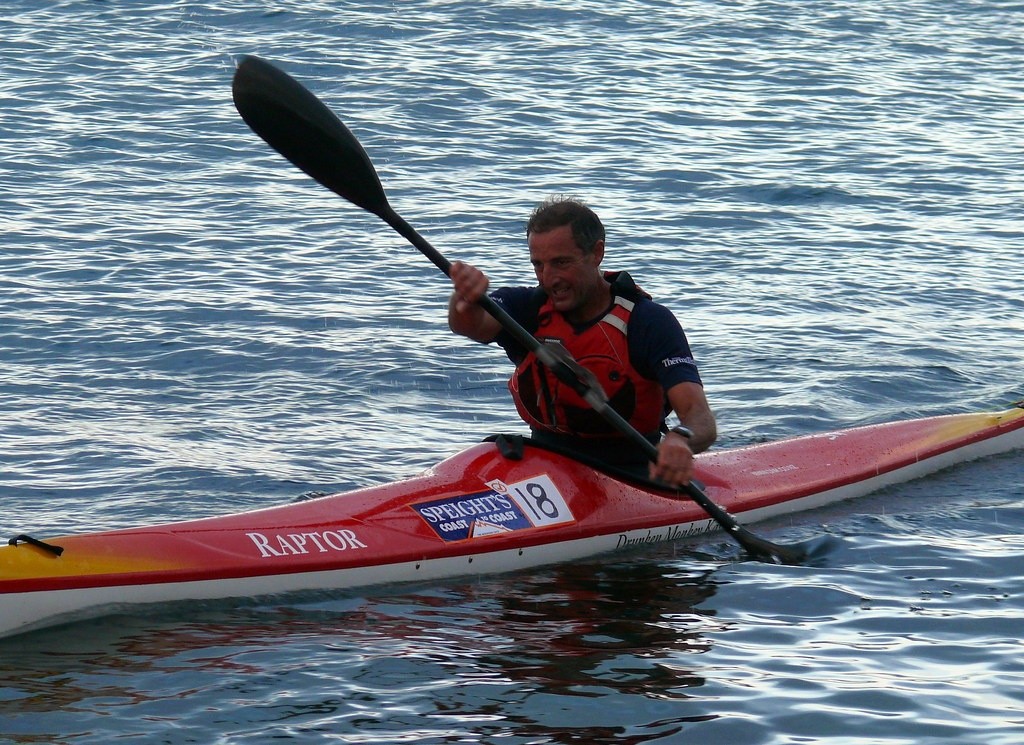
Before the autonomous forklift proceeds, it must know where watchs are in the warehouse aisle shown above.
[671,424,695,438]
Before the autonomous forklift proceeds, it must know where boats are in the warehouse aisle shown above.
[0,405,1024,633]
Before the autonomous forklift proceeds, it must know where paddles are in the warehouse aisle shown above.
[228,51,838,566]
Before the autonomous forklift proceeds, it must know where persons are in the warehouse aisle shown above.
[447,199,717,490]
